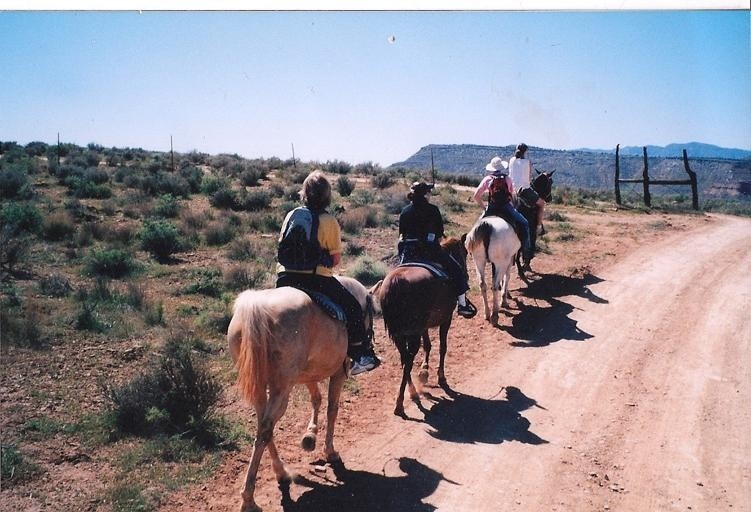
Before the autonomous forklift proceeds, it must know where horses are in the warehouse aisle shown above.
[379,230,468,422]
[491,169,558,292]
[465,185,523,325]
[225,276,385,510]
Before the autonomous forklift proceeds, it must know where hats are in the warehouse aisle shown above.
[488,157,508,173]
[407,183,436,200]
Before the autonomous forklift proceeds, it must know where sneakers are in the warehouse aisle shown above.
[351,358,374,375]
[522,264,536,273]
[537,229,544,235]
[458,305,473,314]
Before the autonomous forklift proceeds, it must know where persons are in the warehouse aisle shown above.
[399,182,474,316]
[475,156,538,274]
[509,144,548,236]
[275,170,380,375]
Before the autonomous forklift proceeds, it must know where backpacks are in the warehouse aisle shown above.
[487,173,513,209]
[277,205,329,270]
